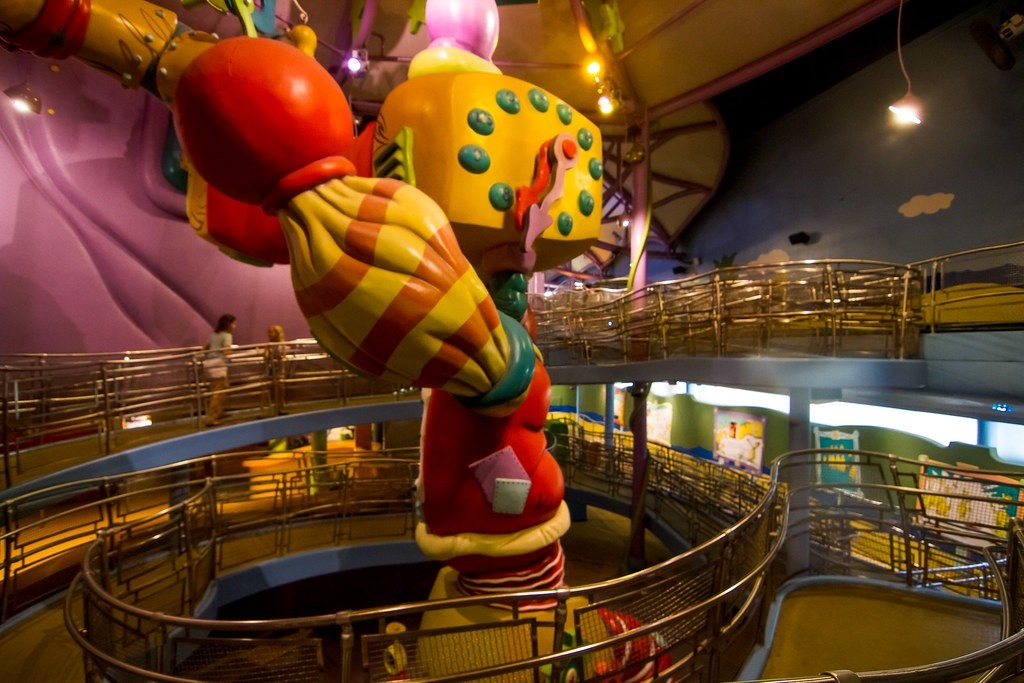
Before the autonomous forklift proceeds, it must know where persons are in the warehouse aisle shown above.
[202,314,237,426]
[264,324,292,414]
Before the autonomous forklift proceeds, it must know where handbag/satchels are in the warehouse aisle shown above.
[203,358,227,378]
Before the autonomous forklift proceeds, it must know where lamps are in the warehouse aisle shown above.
[888,0,925,127]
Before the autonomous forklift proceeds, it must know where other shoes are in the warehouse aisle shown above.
[217,413,233,420]
[278,412,289,416]
[205,421,223,427]
